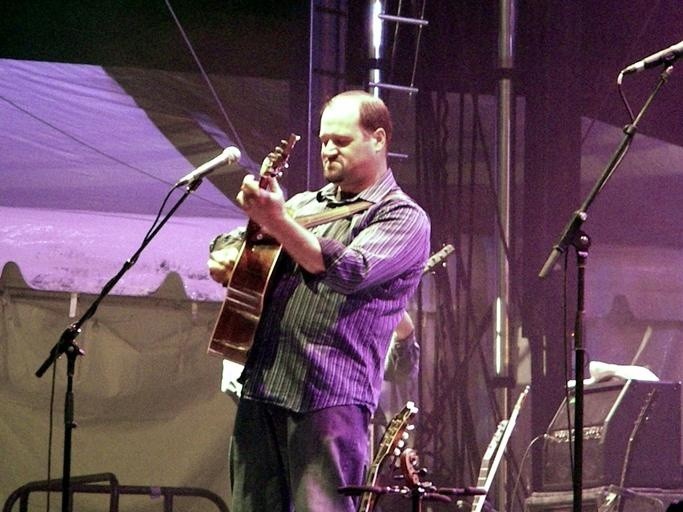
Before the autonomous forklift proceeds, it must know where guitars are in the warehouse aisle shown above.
[206,132,301,369]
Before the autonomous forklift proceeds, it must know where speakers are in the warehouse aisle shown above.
[542,381,683,491]
[526,486,683,512]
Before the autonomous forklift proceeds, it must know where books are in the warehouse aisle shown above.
[563,359,660,390]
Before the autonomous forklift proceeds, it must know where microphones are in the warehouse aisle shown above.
[176,146,241,186]
[622,41,683,74]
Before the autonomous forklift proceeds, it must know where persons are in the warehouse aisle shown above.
[206,91,428,511]
[382,306,420,387]
